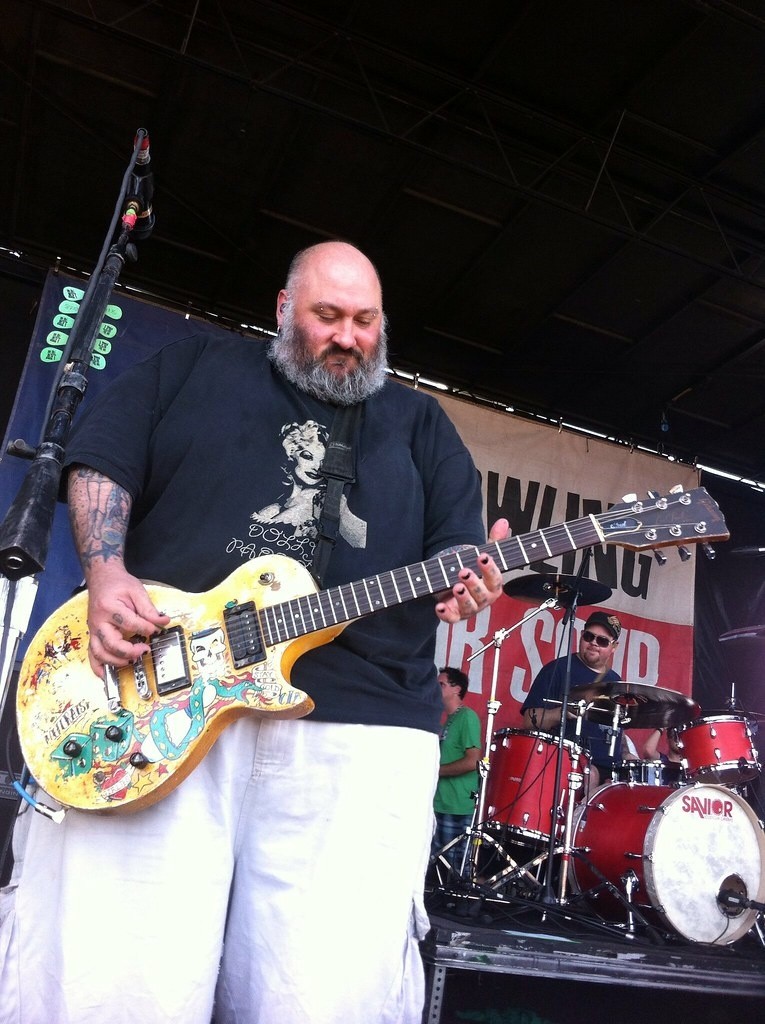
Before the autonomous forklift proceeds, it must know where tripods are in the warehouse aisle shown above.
[427,545,665,948]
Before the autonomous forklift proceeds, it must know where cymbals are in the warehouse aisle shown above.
[701,707,765,723]
[503,571,614,608]
[558,680,704,730]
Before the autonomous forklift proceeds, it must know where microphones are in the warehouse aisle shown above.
[718,889,765,914]
[129,128,154,240]
[532,708,537,724]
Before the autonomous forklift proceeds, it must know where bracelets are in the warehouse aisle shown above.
[656,729,662,736]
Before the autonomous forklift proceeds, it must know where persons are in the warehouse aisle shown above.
[1,240,509,1024]
[621,732,640,760]
[643,728,682,770]
[427,666,481,887]
[520,611,623,799]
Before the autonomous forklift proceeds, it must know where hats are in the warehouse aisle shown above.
[583,611,622,640]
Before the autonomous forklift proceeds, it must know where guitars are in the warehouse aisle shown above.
[13,486,731,814]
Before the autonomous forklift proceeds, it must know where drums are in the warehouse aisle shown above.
[569,782,764,943]
[481,726,590,845]
[610,758,685,789]
[676,713,763,790]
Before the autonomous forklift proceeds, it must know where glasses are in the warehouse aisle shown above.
[582,630,615,648]
